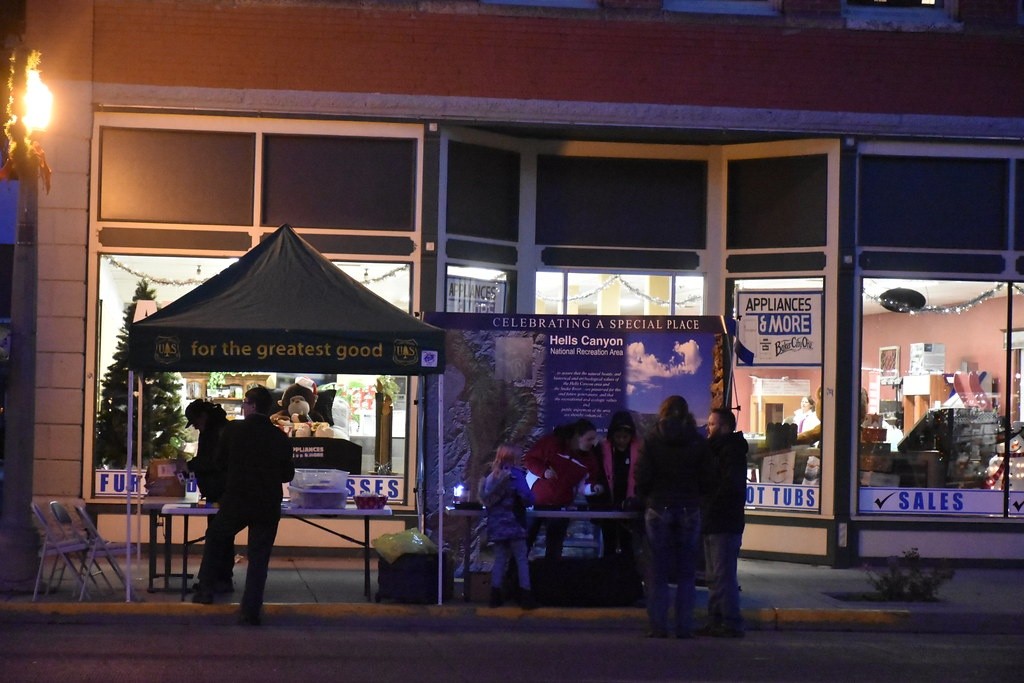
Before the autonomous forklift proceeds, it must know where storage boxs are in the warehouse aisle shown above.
[910,343,945,374]
[287,469,350,510]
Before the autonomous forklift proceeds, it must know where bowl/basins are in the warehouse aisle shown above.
[379,497,388,509]
[353,496,379,509]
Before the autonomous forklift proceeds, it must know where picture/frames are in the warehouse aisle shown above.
[878,345,900,377]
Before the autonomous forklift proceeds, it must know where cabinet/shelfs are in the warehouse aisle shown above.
[180,372,271,415]
[903,375,954,436]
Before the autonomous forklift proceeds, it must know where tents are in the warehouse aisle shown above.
[125,224,446,605]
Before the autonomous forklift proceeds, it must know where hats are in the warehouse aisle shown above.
[184,398,213,428]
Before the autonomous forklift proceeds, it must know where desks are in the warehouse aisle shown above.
[143,497,392,603]
[446,505,643,603]
[890,451,946,487]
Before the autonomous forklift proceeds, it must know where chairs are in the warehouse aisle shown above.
[31,501,137,603]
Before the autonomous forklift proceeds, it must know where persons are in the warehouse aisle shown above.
[507,410,642,586]
[633,396,707,638]
[193,389,295,624]
[691,409,749,639]
[793,396,821,433]
[481,445,536,609]
[186,398,235,592]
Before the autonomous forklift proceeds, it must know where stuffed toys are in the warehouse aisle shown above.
[271,379,335,437]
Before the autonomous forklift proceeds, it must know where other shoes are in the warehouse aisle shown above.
[489,589,506,607]
[191,586,214,604]
[646,630,666,638]
[192,579,235,594]
[520,591,534,610]
[676,630,694,639]
[713,624,744,637]
[695,624,719,636]
[238,607,261,626]
